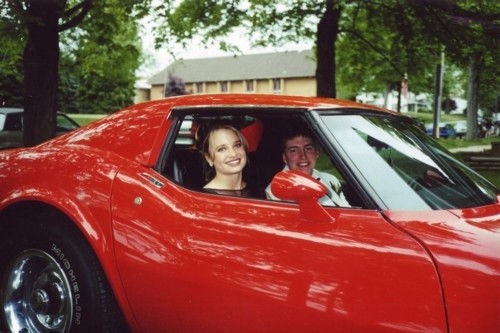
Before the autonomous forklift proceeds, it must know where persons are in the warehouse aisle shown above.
[265,127,351,207]
[201,122,266,198]
[477,117,492,139]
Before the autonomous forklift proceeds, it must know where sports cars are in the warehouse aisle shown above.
[0,93,500,332]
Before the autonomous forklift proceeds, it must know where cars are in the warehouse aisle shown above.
[0,106,81,150]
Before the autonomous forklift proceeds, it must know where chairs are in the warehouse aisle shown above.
[241,152,268,200]
[167,149,204,190]
